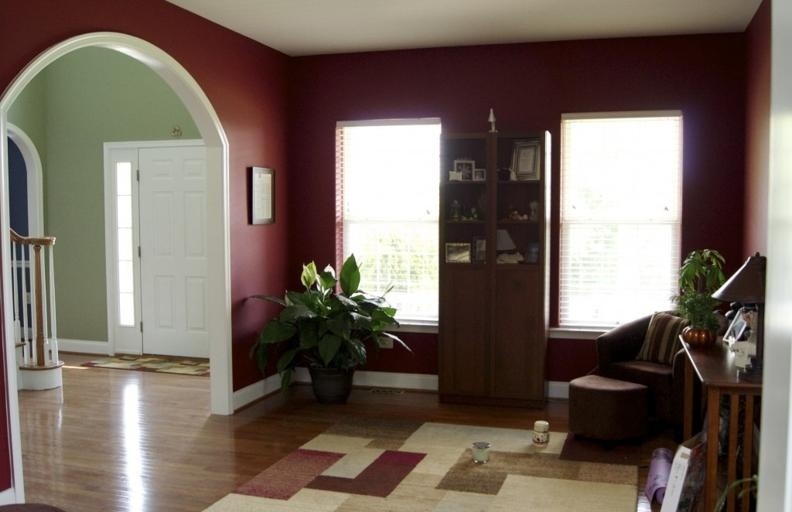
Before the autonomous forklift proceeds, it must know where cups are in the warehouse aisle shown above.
[472,441,490,465]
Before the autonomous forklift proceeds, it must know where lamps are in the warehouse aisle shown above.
[709,250,765,384]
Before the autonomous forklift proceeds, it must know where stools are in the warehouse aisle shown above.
[567,373,649,450]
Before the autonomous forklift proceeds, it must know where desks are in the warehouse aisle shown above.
[677,330,764,511]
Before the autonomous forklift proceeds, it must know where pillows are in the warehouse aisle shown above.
[635,310,692,367]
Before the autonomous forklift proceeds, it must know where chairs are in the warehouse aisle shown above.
[595,309,730,439]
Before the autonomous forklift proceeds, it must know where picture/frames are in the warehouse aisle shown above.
[510,139,541,180]
[452,159,486,181]
[250,164,276,226]
[445,234,488,265]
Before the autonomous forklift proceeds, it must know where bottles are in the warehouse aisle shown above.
[532,420,550,449]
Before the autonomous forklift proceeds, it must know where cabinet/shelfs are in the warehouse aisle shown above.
[437,127,557,411]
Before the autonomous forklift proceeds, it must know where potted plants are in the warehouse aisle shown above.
[669,247,728,350]
[243,252,415,404]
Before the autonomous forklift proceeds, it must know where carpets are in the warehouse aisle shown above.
[198,411,642,512]
[78,354,211,377]
